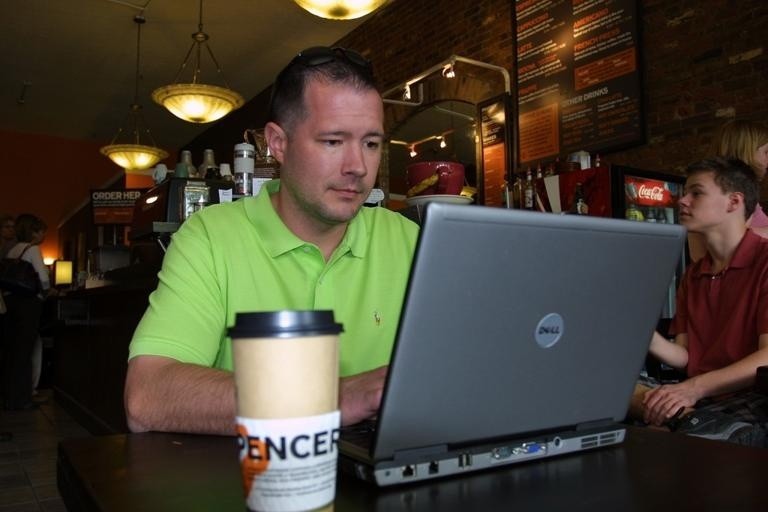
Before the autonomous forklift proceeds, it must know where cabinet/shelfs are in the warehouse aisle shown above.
[56,195,135,280]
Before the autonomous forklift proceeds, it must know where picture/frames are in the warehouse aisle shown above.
[510,0,647,174]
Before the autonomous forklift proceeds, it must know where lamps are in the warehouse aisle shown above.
[42,257,58,265]
[402,86,411,101]
[440,136,447,149]
[98,14,170,173]
[410,144,418,158]
[54,260,73,286]
[292,0,392,22]
[441,64,456,79]
[148,0,246,125]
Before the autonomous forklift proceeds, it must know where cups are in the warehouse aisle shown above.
[152,164,168,186]
[404,161,465,198]
[175,163,189,177]
[223,309,346,512]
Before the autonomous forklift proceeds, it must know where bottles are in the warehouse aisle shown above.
[199,148,219,179]
[533,160,545,212]
[232,142,255,203]
[180,150,198,177]
[87,249,95,276]
[523,163,535,211]
[205,168,220,205]
[213,163,235,205]
[566,150,591,170]
[645,207,656,223]
[657,207,667,224]
[626,204,645,222]
[572,182,589,215]
[594,153,601,167]
[512,174,525,210]
[501,173,514,208]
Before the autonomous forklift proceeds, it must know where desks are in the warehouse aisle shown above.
[56,427,768,512]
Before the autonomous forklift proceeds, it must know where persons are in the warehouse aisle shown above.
[31,217,49,403]
[0,215,17,257]
[688,119,768,263]
[628,156,768,447]
[122,46,422,436]
[0,214,50,410]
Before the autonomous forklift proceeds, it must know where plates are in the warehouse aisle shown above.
[405,194,474,207]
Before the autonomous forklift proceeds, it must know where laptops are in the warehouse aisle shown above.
[339,201,686,490]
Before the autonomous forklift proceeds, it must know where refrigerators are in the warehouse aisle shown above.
[533,164,692,379]
